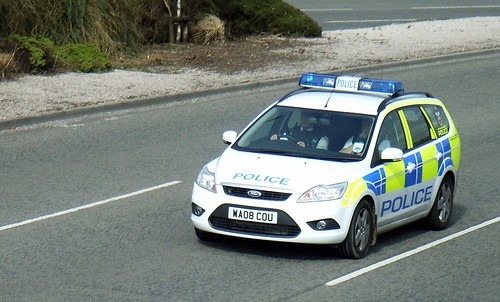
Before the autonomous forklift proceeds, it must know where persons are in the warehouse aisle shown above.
[271,110,330,155]
[338,115,394,167]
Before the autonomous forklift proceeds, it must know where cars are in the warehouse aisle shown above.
[191,72,462,259]
